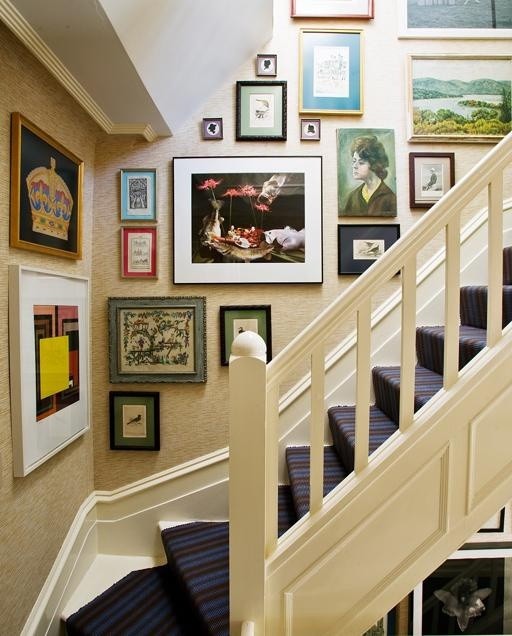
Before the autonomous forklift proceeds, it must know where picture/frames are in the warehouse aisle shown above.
[361,507,511,635]
[10,111,84,260]
[9,264,91,478]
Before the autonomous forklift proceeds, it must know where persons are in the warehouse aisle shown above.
[343,136,396,216]
[426,168,438,191]
